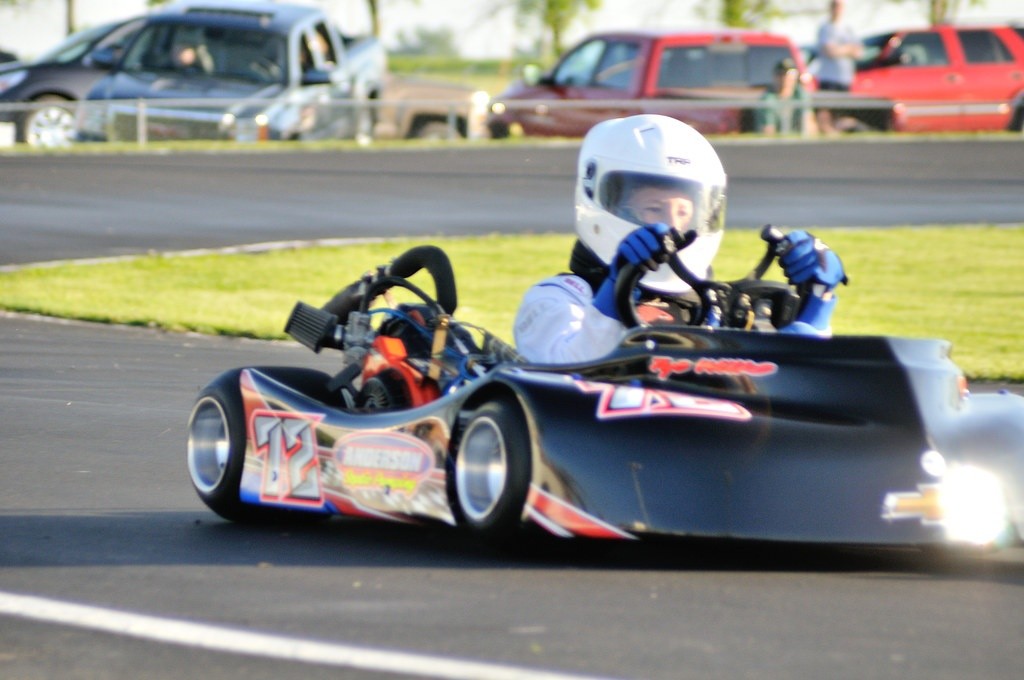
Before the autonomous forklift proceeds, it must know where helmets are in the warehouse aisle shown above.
[573,113,727,294]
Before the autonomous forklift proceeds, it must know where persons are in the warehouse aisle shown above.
[815,0,862,134]
[514,112,845,381]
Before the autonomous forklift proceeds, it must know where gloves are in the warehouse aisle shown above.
[772,230,848,301]
[609,221,697,284]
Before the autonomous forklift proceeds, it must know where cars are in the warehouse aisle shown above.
[74,1,389,145]
[483,25,815,143]
[0,14,156,151]
[833,23,1024,134]
